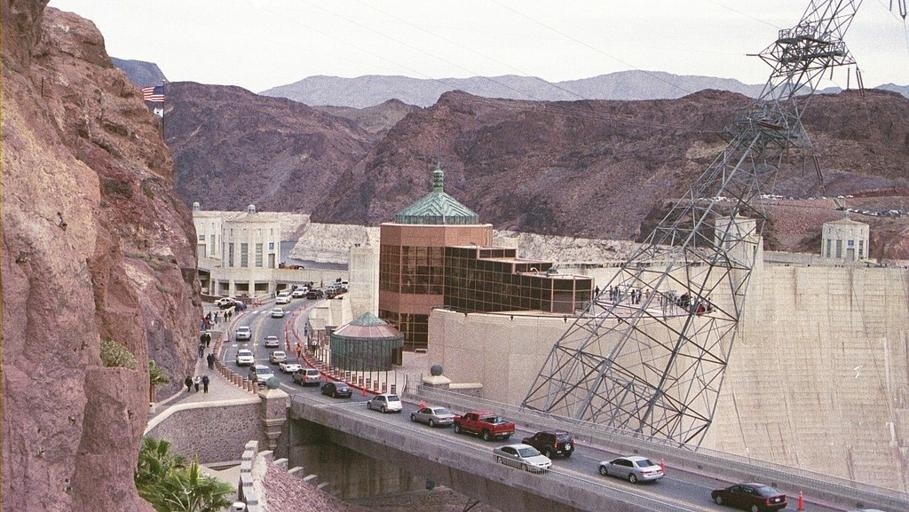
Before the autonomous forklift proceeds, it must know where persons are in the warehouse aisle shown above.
[609,285,718,316]
[304,323,309,336]
[594,285,600,298]
[182,309,232,392]
[296,344,303,358]
[272,288,280,298]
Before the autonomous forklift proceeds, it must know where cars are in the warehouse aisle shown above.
[594,454,664,486]
[707,192,858,203]
[320,379,354,398]
[834,204,899,217]
[704,482,789,512]
[410,406,456,428]
[214,279,352,388]
[490,442,554,474]
[366,392,404,415]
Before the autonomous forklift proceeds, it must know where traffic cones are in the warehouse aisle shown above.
[658,455,666,475]
[795,490,806,512]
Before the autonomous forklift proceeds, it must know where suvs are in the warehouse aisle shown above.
[520,429,576,461]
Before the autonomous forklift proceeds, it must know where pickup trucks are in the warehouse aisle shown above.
[450,410,516,443]
